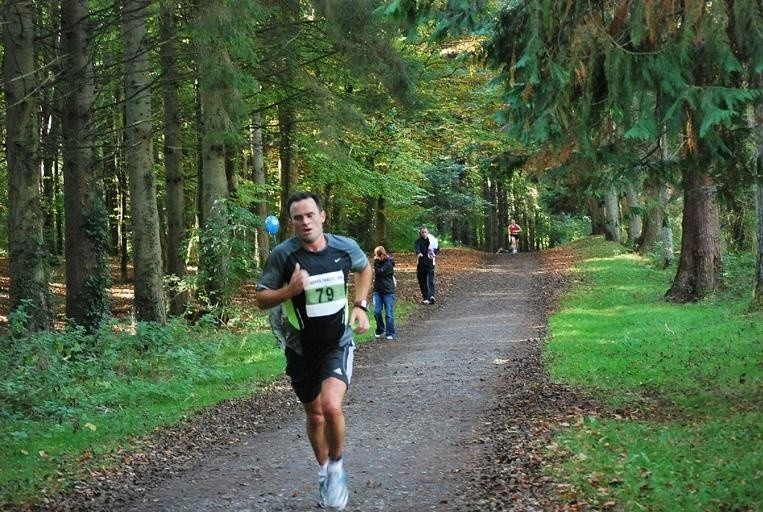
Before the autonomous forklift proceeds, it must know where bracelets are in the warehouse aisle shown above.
[352,298,370,311]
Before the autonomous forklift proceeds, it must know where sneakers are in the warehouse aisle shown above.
[386,336,393,340]
[326,466,348,512]
[421,300,429,304]
[317,469,328,509]
[429,296,436,303]
[374,333,381,339]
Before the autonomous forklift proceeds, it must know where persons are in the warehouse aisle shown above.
[507,219,522,253]
[414,226,440,304]
[372,244,396,340]
[254,191,374,511]
